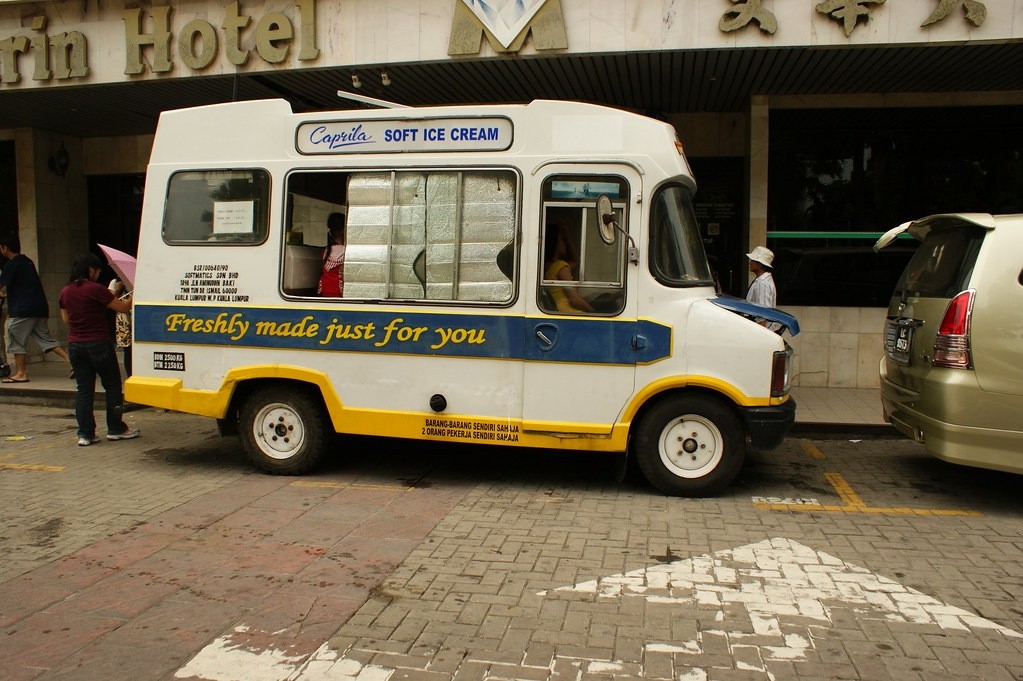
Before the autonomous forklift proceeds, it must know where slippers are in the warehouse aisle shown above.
[2,377,29,382]
[69,369,75,378]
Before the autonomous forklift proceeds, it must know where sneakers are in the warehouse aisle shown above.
[107,428,140,440]
[78,435,99,446]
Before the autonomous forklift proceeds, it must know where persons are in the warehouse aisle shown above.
[545,216,622,313]
[108,276,132,377]
[746,246,777,331]
[58,250,140,446]
[317,212,345,298]
[0,233,74,382]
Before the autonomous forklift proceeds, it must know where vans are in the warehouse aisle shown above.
[873,210,1023,478]
[121,91,803,498]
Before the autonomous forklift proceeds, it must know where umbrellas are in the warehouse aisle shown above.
[97,243,136,299]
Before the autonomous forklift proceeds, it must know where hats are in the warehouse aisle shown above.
[746,246,775,269]
